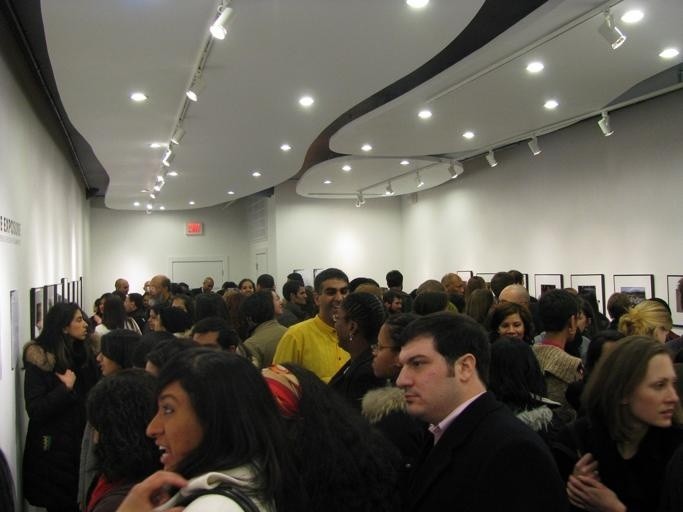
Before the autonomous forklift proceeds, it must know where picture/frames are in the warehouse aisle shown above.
[456,271,528,296]
[667,275,683,326]
[534,274,563,300]
[613,274,654,299]
[570,274,605,315]
[31,277,82,338]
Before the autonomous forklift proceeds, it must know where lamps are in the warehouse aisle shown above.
[385,181,394,196]
[529,136,541,155]
[357,192,365,204]
[447,164,460,179]
[425,0,626,103]
[415,173,425,189]
[598,111,617,138]
[486,151,501,172]
[145,0,236,214]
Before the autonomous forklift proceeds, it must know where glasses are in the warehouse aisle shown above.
[368,339,397,352]
[331,313,354,324]
